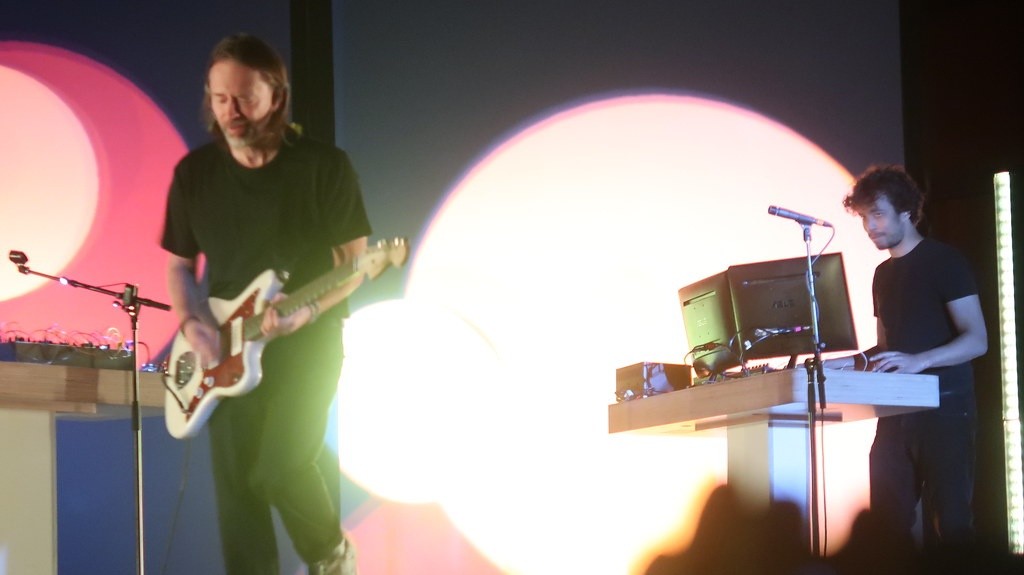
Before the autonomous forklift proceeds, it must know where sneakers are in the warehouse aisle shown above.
[308,537,357,575]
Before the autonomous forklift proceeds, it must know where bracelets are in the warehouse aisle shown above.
[179,316,201,337]
[308,302,319,324]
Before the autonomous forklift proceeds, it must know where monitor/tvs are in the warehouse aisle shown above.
[678,252,859,380]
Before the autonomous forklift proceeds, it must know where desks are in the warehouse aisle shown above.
[608,368,940,521]
[0,362,167,575]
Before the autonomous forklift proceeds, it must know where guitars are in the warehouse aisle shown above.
[157,236,415,441]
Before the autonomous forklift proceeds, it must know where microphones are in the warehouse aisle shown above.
[768,206,832,228]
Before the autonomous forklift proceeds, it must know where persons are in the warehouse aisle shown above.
[798,165,988,553]
[157,35,373,574]
[645,485,1024,574]
